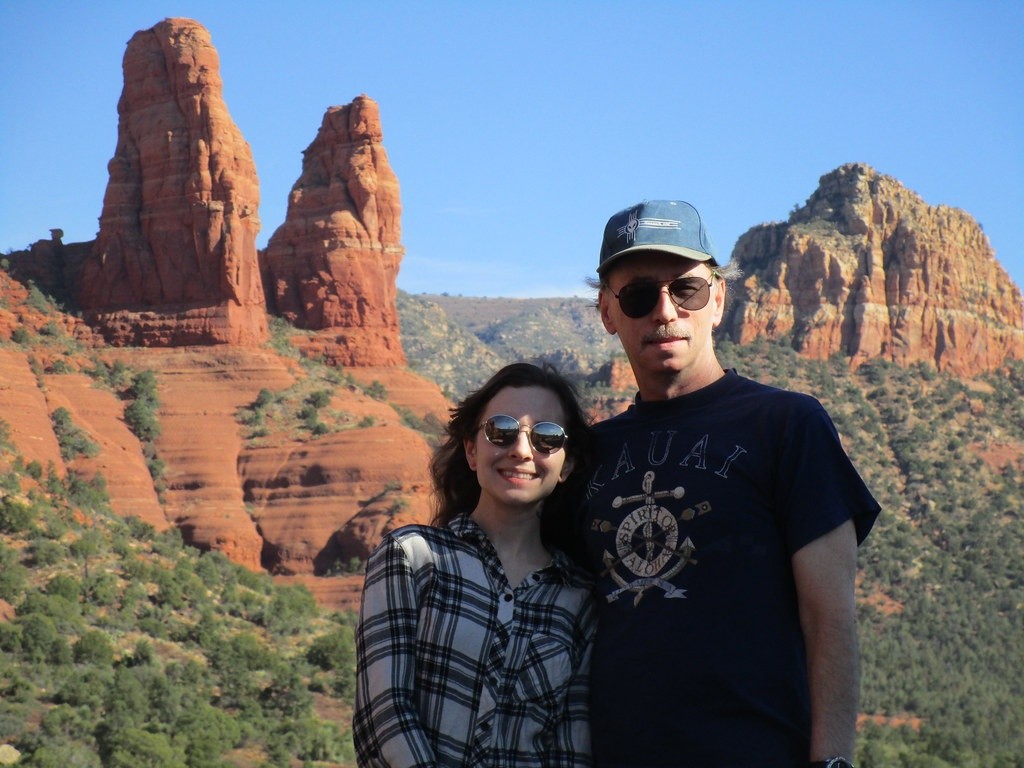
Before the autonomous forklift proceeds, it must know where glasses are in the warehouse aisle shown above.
[604,272,715,320]
[477,414,569,456]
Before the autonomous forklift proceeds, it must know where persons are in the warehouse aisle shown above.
[540,202,882,768]
[352,362,601,768]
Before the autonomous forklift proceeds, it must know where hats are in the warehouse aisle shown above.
[596,200,721,272]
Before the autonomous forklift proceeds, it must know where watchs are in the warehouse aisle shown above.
[810,753,856,768]
